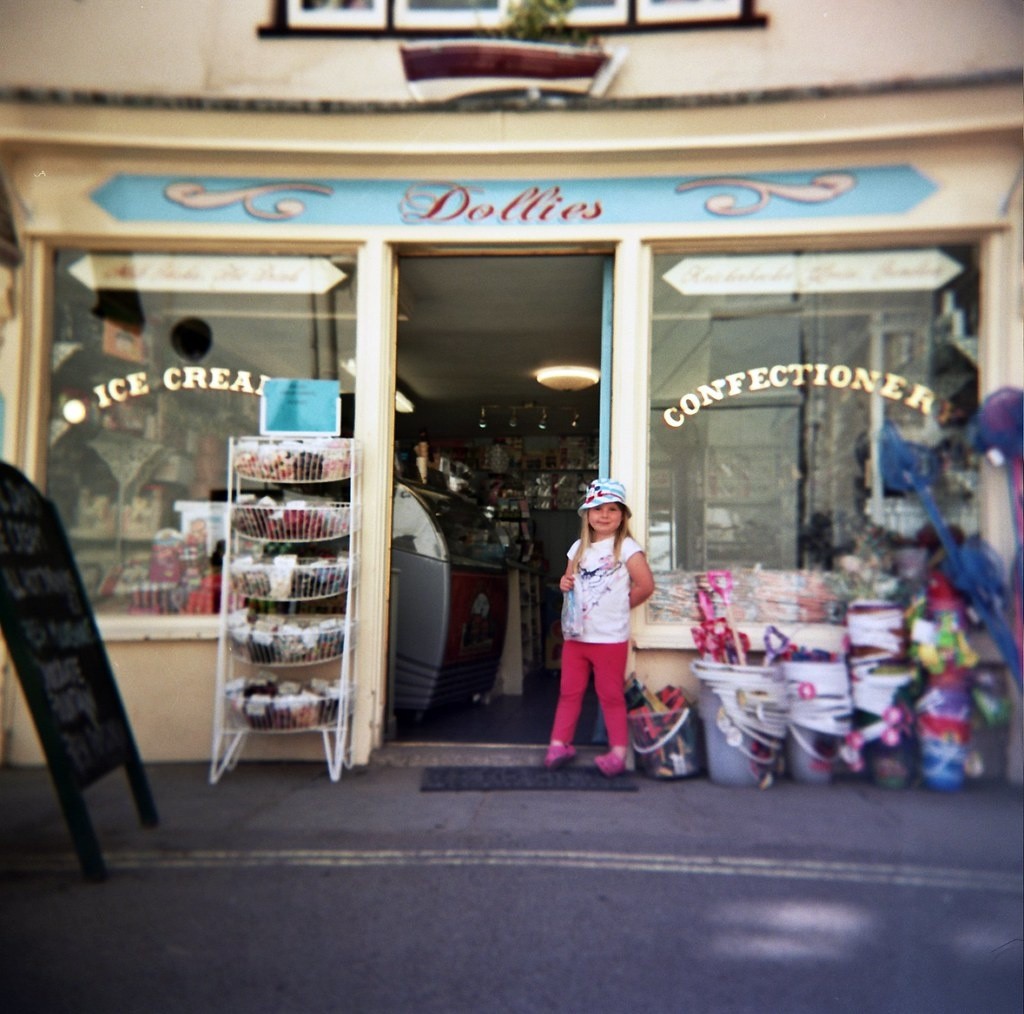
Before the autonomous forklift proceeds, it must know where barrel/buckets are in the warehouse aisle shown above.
[626,706,701,779]
[691,600,971,794]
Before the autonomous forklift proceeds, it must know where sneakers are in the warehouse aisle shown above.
[592,752,625,778]
[544,743,578,772]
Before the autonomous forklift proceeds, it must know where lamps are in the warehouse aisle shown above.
[477,404,581,430]
[535,364,601,392]
[337,352,414,412]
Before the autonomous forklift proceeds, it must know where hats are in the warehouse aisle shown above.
[578,478,631,517]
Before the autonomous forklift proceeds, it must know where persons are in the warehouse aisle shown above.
[544,478,654,777]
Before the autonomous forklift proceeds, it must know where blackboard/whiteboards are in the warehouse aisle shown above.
[1,465,147,795]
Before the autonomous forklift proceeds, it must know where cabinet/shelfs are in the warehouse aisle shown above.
[691,444,793,571]
[646,461,674,572]
[204,434,361,786]
[480,465,599,523]
[44,302,190,608]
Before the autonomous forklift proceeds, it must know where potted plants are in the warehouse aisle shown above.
[399,0,614,104]
